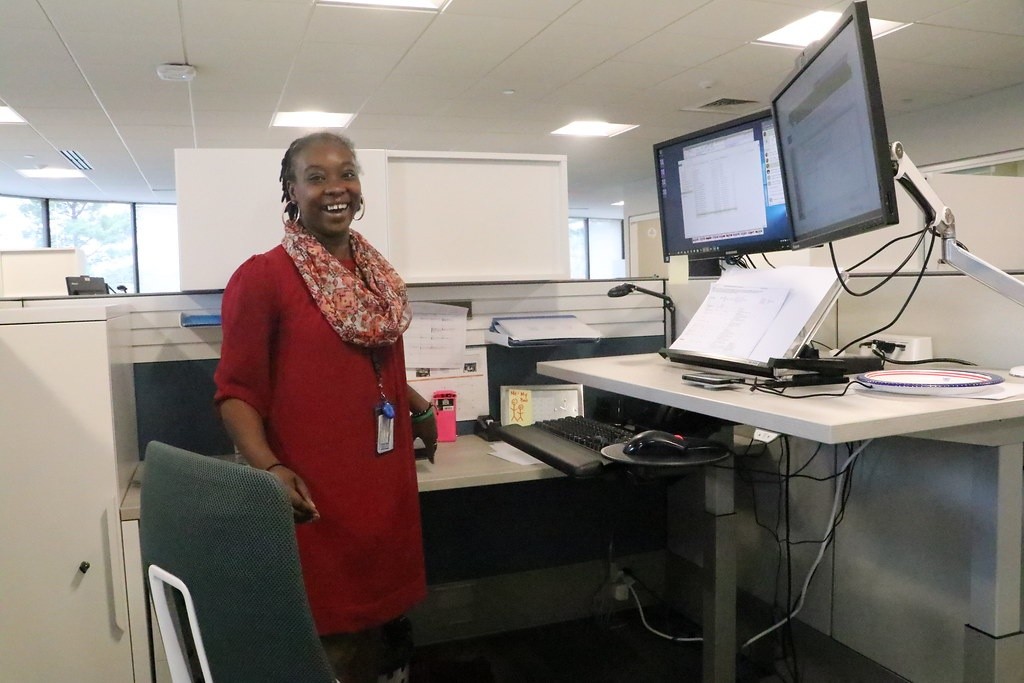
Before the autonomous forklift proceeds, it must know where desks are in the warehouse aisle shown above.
[536,349,1024,683]
[115,417,739,683]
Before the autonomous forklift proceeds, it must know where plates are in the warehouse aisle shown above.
[857,368,1005,396]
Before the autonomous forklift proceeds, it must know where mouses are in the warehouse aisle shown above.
[623,429,689,456]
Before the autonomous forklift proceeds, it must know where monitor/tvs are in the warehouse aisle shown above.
[653,107,796,263]
[771,0,899,250]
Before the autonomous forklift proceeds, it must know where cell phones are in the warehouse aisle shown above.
[680,372,745,385]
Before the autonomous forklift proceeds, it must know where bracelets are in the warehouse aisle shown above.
[411,403,434,422]
[264,462,287,475]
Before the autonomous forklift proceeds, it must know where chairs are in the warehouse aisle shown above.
[140,439,341,683]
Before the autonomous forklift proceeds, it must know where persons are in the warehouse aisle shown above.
[215,129,437,683]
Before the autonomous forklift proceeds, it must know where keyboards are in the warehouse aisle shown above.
[500,414,642,480]
